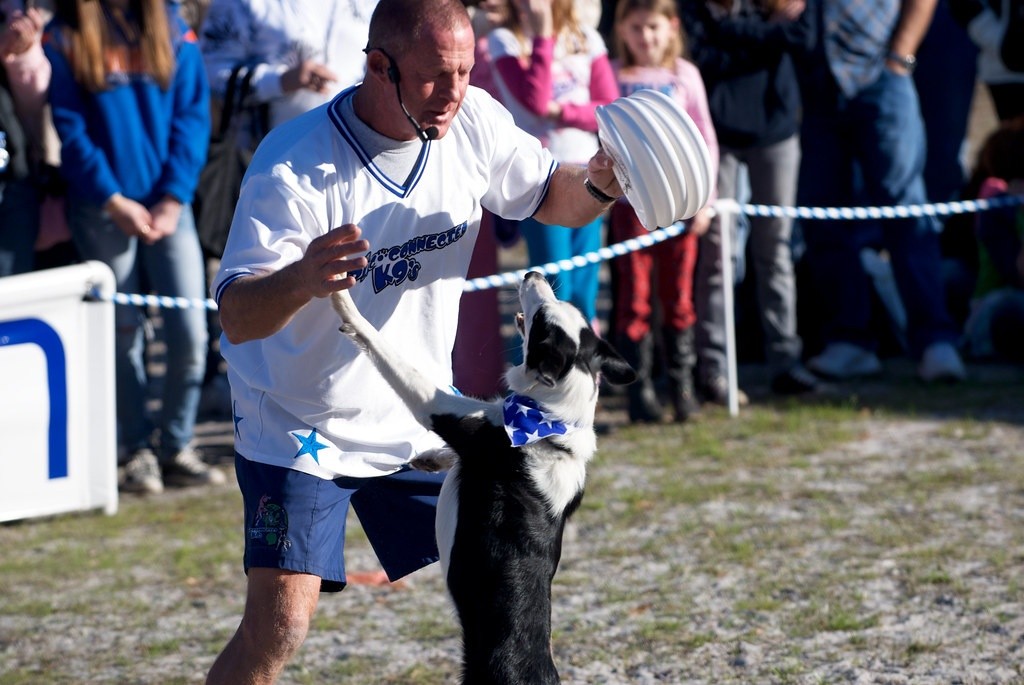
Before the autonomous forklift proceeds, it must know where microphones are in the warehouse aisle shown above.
[387,61,439,143]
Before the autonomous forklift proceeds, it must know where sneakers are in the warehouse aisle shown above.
[806,342,884,383]
[162,442,228,488]
[698,376,750,410]
[118,448,167,495]
[772,367,837,400]
[914,344,968,400]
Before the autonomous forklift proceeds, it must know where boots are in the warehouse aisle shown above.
[605,322,696,424]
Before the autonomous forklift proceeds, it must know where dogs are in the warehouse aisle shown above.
[324,237,636,685]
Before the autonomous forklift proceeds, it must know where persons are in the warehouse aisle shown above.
[608,0,720,422]
[791,0,965,379]
[483,0,621,431]
[676,1,844,406]
[0,0,1024,427]
[204,0,625,685]
[42,1,226,494]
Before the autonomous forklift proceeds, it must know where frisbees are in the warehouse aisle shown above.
[636,88,715,209]
[594,104,659,235]
[604,101,676,231]
[627,93,706,220]
[614,94,687,221]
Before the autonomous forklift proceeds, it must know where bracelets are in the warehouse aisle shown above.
[887,49,916,70]
[584,178,615,204]
